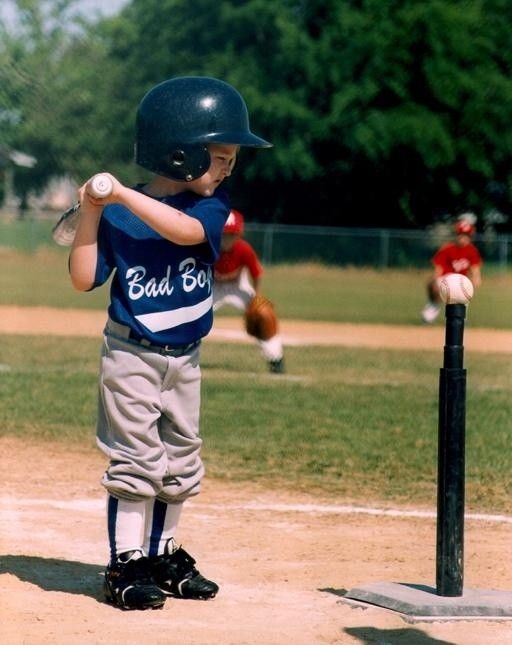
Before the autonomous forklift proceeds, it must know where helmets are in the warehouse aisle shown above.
[131,75,275,183]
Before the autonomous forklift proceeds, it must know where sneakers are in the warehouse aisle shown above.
[421,302,441,323]
[269,360,284,373]
[103,558,167,611]
[148,546,219,600]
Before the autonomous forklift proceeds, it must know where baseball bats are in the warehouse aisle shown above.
[53,174,112,245]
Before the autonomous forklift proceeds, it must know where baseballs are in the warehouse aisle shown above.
[440,273,474,305]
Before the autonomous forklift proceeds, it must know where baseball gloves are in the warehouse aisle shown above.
[246,293,275,340]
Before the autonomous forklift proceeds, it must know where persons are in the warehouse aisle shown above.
[202,208,289,376]
[417,218,482,324]
[65,76,275,613]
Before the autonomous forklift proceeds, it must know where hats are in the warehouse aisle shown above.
[455,220,476,238]
[221,209,246,236]
